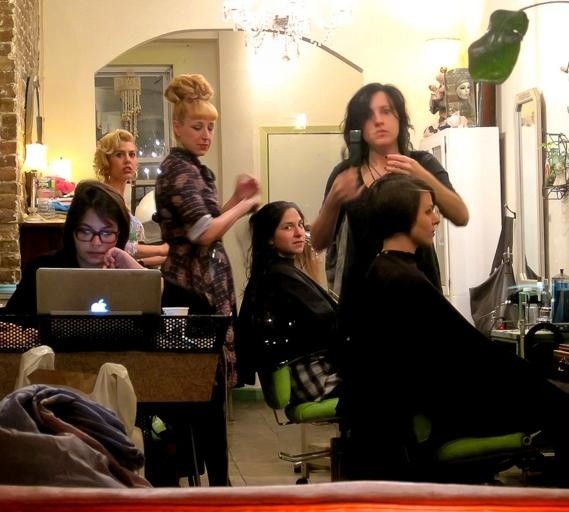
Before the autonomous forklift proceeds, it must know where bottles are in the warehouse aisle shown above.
[38,174,56,219]
[528,279,552,329]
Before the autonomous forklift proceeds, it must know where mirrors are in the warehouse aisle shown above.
[511,88,546,290]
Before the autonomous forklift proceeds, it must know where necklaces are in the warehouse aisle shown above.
[367,163,381,183]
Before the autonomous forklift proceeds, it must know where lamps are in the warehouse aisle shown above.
[219,1,355,65]
[21,144,51,224]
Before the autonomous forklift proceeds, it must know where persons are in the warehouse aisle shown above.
[1,180,209,314]
[154,74,262,390]
[94,129,170,267]
[310,83,470,295]
[337,174,568,488]
[248,201,338,401]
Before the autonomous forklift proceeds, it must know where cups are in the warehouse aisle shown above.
[161,307,190,341]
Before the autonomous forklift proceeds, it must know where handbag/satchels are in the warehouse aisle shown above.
[467,215,523,337]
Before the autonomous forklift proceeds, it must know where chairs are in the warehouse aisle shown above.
[253,362,338,485]
[386,388,536,485]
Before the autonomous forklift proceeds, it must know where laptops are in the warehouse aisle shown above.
[36,268,161,316]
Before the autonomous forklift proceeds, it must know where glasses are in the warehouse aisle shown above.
[65,224,122,244]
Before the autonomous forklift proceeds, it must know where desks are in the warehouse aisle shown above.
[2,345,215,487]
